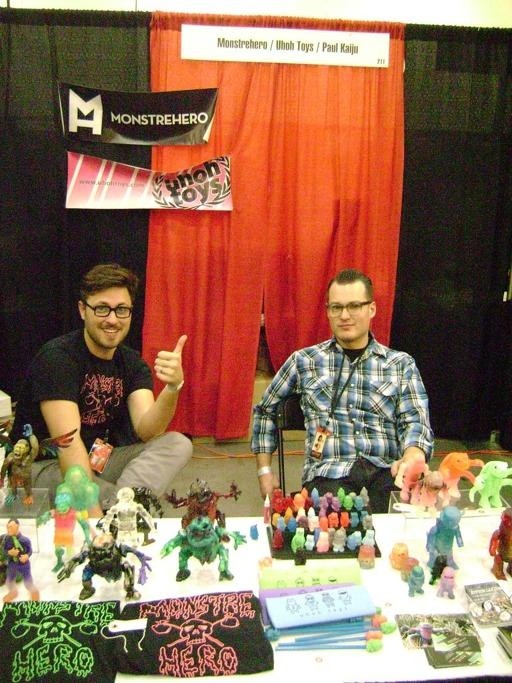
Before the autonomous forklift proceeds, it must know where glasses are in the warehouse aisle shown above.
[327,301,373,317]
[86,303,132,319]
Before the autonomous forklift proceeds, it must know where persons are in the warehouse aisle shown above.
[5,263,194,517]
[436,566,457,600]
[0,518,40,601]
[250,270,434,512]
[429,555,449,585]
[426,505,464,571]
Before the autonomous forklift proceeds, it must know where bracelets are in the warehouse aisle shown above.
[168,382,185,394]
[257,466,272,477]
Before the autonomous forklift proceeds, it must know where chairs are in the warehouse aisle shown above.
[274,391,305,503]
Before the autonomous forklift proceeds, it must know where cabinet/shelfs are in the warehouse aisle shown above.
[1,504,512,682]
